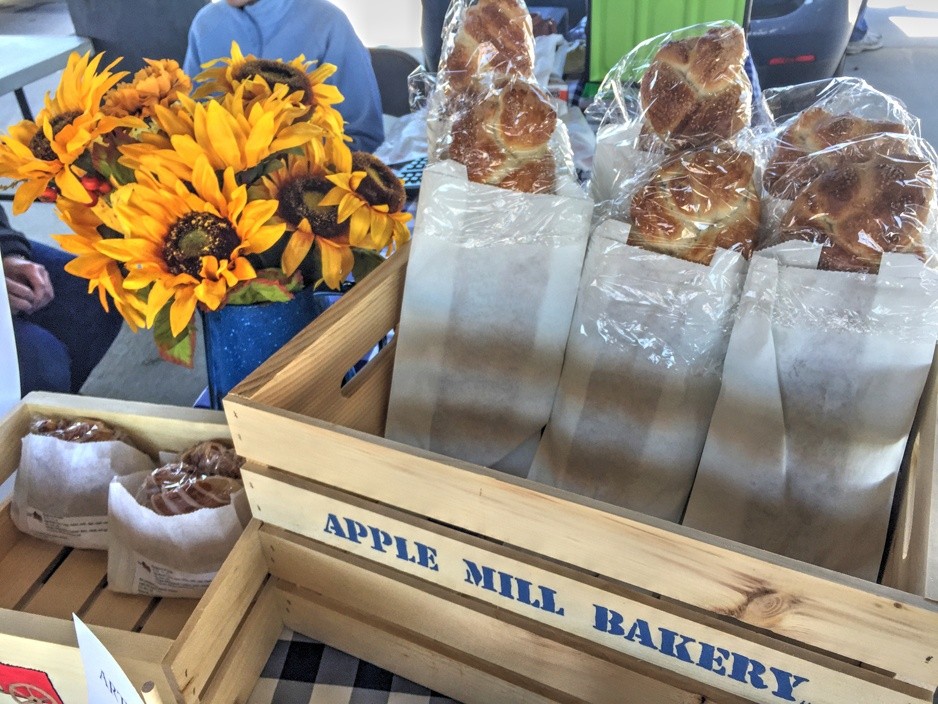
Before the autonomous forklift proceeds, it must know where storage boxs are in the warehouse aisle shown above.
[219,231,935,704]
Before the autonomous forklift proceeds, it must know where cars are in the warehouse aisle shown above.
[417,1,863,96]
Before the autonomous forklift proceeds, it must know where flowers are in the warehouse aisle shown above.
[0,55,411,368]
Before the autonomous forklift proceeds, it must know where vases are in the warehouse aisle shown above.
[195,297,316,412]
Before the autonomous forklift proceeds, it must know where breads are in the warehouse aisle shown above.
[136,440,245,515]
[761,108,935,275]
[439,1,557,194]
[29,417,135,448]
[625,23,761,266]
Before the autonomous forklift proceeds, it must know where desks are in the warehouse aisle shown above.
[0,35,95,204]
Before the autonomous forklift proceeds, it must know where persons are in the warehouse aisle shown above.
[846,0,883,53]
[181,0,386,155]
[0,202,126,401]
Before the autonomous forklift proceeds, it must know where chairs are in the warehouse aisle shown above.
[365,47,426,121]
